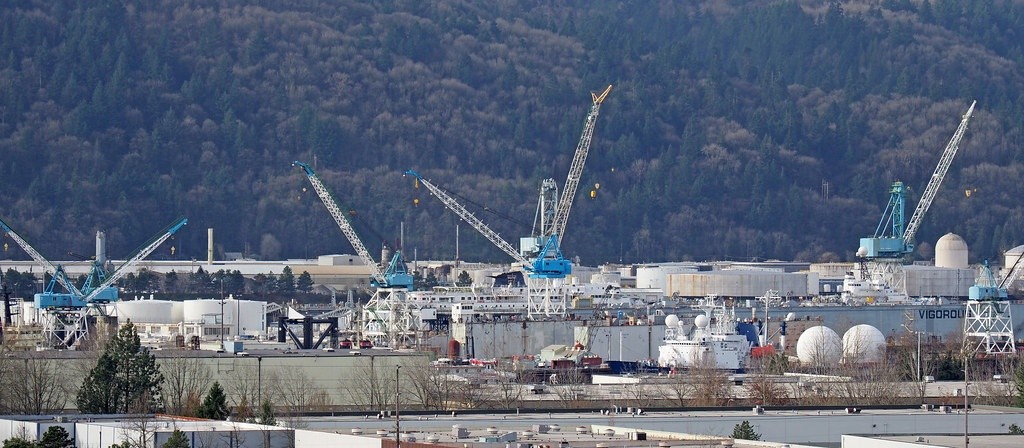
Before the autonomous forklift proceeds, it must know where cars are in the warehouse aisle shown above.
[339,338,373,349]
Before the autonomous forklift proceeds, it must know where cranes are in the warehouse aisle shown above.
[959,250,1024,360]
[857,97,978,296]
[0,221,121,336]
[566,285,616,370]
[402,169,559,280]
[290,159,415,350]
[527,84,613,323]
[35,219,189,350]
